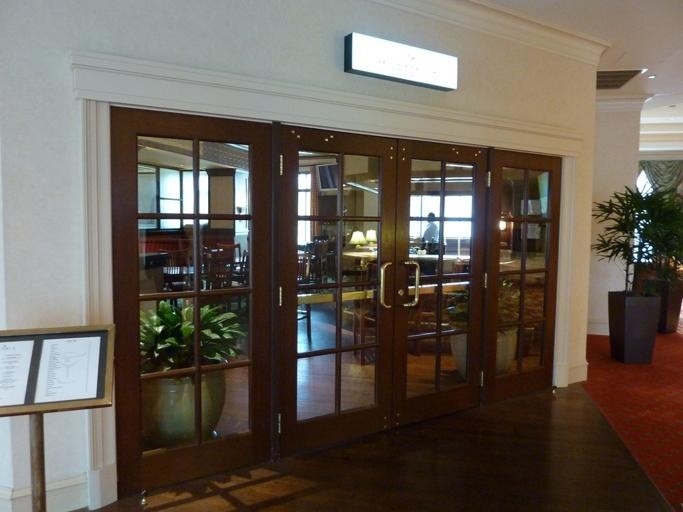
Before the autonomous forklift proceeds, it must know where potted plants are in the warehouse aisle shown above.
[141,301,245,452]
[592,187,658,365]
[641,187,683,333]
[445,278,520,384]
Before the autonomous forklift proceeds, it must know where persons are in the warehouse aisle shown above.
[422,213,445,255]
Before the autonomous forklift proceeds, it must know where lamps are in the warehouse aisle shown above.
[500,210,516,252]
[365,230,377,251]
[347,229,366,251]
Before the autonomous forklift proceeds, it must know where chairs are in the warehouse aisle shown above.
[351,260,474,364]
[164,243,326,338]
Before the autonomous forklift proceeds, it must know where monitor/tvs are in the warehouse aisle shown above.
[316,164,350,191]
